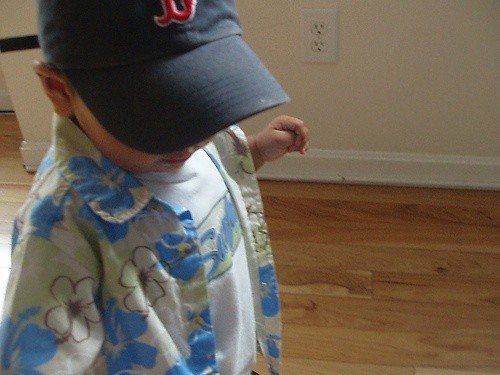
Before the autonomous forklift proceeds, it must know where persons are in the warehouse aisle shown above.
[0,0,310,375]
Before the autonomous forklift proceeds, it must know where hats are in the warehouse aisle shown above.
[36,0,292,153]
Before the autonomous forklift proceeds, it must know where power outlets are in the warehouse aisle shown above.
[299,9,340,65]
